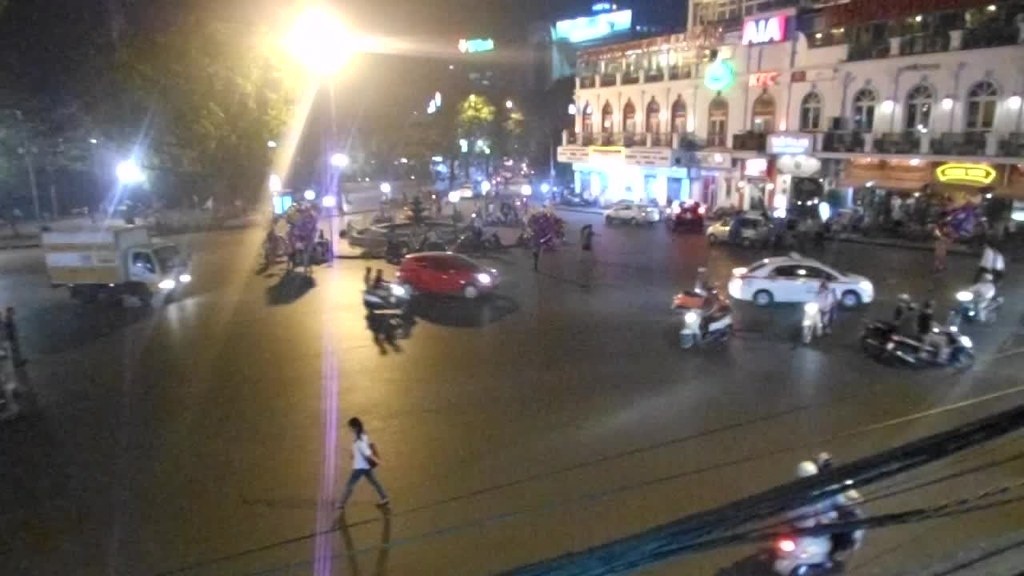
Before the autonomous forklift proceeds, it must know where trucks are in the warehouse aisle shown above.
[40,222,191,302]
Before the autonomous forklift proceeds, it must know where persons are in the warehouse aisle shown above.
[2,307,29,369]
[333,415,390,510]
[693,264,715,311]
[881,212,1008,367]
[816,280,834,334]
[791,446,858,549]
[698,283,722,336]
[268,188,527,309]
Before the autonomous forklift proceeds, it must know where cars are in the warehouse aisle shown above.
[0,332,28,425]
[664,199,704,231]
[705,215,767,248]
[728,251,874,312]
[606,203,661,226]
[398,251,501,299]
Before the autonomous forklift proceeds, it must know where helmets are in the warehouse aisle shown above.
[897,292,910,307]
[795,460,818,478]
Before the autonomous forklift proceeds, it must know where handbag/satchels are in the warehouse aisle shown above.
[368,443,378,467]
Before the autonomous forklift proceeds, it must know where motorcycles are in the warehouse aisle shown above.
[859,290,1002,370]
[803,293,835,344]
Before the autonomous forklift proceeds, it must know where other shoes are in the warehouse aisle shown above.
[374,496,389,507]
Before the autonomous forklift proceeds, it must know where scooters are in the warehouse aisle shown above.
[772,451,866,575]
[382,240,410,265]
[360,278,411,309]
[679,299,734,350]
[478,230,505,256]
